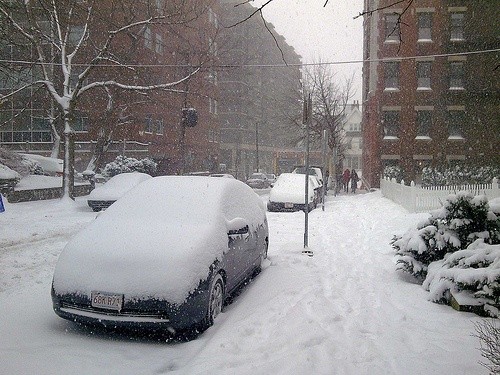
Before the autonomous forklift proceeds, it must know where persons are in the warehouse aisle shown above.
[343,168,350,193]
[325,171,329,195]
[350,169,358,193]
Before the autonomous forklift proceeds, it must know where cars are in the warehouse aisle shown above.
[267,166,323,212]
[88,172,153,211]
[51,172,270,342]
[246,173,269,189]
